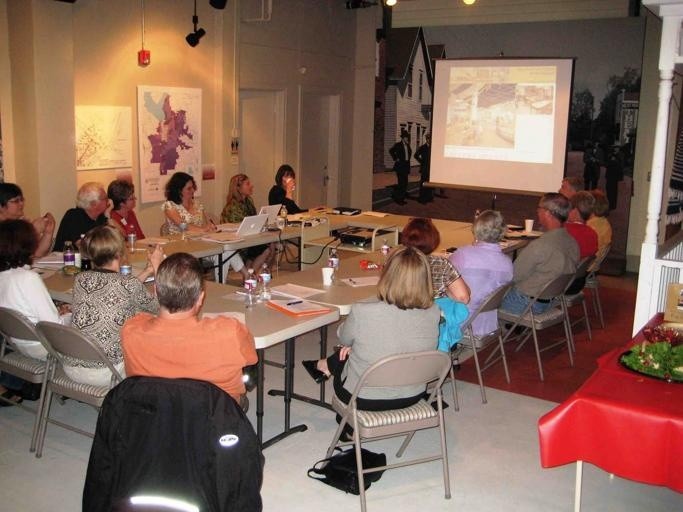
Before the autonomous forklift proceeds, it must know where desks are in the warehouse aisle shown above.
[537,298,683,512]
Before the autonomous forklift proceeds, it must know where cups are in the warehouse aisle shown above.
[322,268,335,287]
[524,219,535,232]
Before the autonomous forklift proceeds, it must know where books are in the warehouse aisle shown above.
[35,255,65,265]
[209,232,241,241]
[200,235,245,245]
[332,206,360,216]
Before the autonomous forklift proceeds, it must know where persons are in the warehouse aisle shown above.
[119,252,258,416]
[0,219,74,361]
[220,173,275,283]
[107,180,146,241]
[268,164,327,246]
[596,130,624,211]
[562,191,599,295]
[388,130,412,206]
[498,191,580,336]
[61,225,167,388]
[445,209,514,373]
[584,189,612,277]
[558,175,583,200]
[300,246,442,442]
[0,182,55,260]
[431,187,449,198]
[583,130,604,191]
[0,383,25,408]
[414,133,433,205]
[400,217,471,305]
[160,172,264,288]
[51,181,114,253]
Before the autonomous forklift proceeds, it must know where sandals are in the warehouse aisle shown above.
[452,357,460,372]
[0,390,23,406]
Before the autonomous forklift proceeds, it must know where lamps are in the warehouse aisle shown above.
[185,0,207,48]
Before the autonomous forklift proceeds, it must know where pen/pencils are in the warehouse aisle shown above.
[149,244,156,248]
[287,301,303,305]
[208,216,217,230]
[349,278,356,284]
[326,212,336,215]
[37,272,44,274]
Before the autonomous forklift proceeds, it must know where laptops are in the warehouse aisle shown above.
[232,214,269,236]
[258,203,282,226]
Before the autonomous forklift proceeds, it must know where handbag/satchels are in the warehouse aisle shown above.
[307,446,386,495]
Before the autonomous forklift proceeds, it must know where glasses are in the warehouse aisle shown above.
[8,197,24,203]
[536,205,544,209]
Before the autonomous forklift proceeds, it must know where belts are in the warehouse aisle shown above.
[530,296,550,303]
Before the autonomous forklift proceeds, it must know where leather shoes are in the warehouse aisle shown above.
[505,324,523,334]
[302,360,329,384]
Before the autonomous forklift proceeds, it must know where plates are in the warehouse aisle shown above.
[619,351,683,385]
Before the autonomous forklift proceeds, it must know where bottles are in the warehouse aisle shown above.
[63,241,75,265]
[179,215,188,244]
[244,268,257,309]
[258,262,271,302]
[118,254,132,276]
[381,238,389,265]
[78,234,91,272]
[328,248,339,285]
[127,223,136,253]
[280,205,288,228]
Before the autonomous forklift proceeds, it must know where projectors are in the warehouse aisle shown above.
[340,229,381,245]
